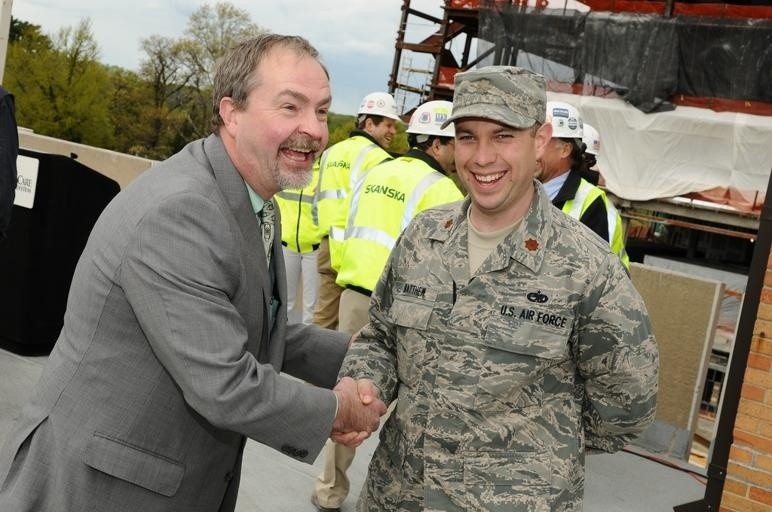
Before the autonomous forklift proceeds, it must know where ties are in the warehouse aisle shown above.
[257,199,278,275]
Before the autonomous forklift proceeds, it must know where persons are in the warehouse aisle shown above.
[274,149,320,329]
[311,97,474,512]
[0,34,387,511]
[335,64,663,512]
[573,122,604,183]
[314,92,402,329]
[531,97,631,280]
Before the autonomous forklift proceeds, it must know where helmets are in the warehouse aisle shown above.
[544,101,583,139]
[405,100,456,138]
[359,91,403,123]
[579,123,600,156]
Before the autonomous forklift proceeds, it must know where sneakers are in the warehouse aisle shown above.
[310,493,342,512]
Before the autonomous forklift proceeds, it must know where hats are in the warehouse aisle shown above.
[441,64,548,130]
